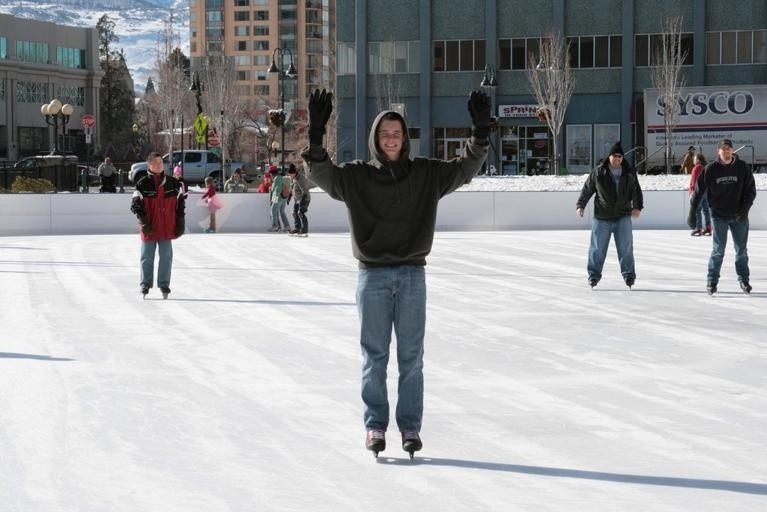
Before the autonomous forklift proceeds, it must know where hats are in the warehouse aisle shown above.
[609,144,624,157]
[288,163,296,174]
[268,165,277,172]
[718,138,734,149]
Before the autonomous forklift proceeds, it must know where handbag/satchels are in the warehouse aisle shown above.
[280,185,288,199]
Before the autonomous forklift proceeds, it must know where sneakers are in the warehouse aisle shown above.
[589,278,597,286]
[623,275,634,286]
[139,282,149,294]
[267,222,309,238]
[706,281,717,292]
[738,277,752,294]
[401,428,422,452]
[205,227,215,233]
[691,228,712,236]
[365,428,386,451]
[158,280,170,294]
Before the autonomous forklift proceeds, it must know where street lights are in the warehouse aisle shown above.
[132,122,147,162]
[41,99,72,155]
[479,63,501,176]
[168,107,175,176]
[188,70,204,149]
[270,139,279,173]
[267,48,299,175]
[219,110,227,192]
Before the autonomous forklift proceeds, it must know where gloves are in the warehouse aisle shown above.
[137,216,153,237]
[174,216,185,237]
[466,90,493,138]
[308,87,333,138]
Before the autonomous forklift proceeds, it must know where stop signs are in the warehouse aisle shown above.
[81,115,95,130]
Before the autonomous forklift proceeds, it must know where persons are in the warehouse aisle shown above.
[690,139,756,293]
[574,141,643,285]
[682,146,696,174]
[304,88,492,451]
[688,154,711,235]
[131,152,185,294]
[97,152,310,236]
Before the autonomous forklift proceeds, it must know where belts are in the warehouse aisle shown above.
[358,258,428,268]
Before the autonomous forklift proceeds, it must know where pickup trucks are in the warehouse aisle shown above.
[127,149,242,190]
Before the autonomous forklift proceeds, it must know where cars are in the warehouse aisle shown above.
[13,156,97,177]
